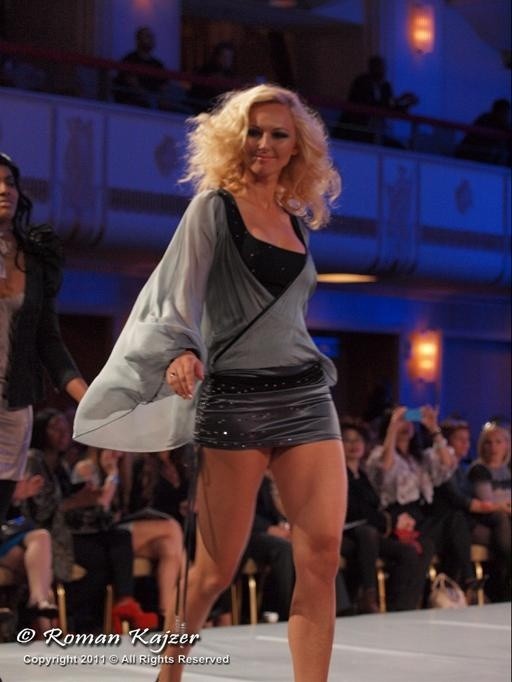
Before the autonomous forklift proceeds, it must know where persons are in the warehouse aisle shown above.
[1,152,90,517]
[110,26,191,110]
[457,98,512,165]
[187,43,245,118]
[333,55,416,150]
[71,81,351,681]
[0,401,512,641]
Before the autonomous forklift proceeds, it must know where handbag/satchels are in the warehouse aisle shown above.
[430,589,465,610]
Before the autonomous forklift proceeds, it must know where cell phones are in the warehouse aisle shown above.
[404,410,424,421]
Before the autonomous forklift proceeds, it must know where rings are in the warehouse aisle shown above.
[166,371,176,377]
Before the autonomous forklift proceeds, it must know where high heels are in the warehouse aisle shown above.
[111,601,158,633]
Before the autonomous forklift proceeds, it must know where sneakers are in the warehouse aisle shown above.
[28,595,58,614]
